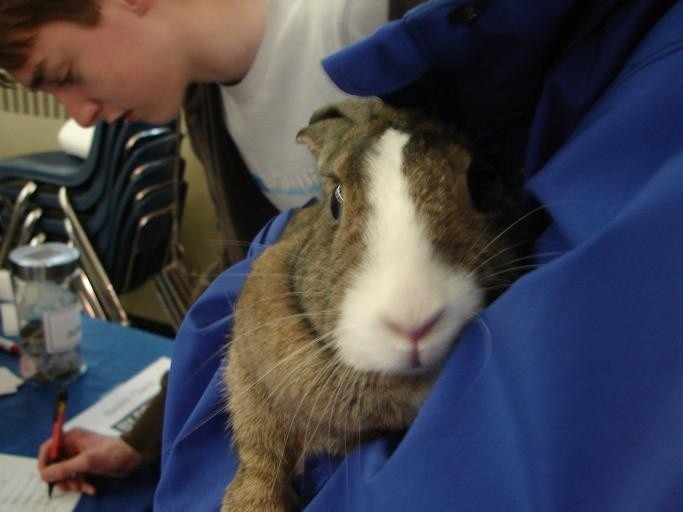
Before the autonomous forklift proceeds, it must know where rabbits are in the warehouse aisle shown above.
[194,95,578,511]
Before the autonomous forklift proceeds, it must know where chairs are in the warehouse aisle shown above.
[0,112,199,336]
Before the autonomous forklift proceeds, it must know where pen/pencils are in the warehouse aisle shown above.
[47,388,68,497]
[0,335,20,354]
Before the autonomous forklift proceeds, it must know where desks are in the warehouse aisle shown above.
[0,310,178,512]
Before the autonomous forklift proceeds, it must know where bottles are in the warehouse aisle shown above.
[7,241,89,391]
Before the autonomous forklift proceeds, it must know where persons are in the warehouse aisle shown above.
[0,1,403,496]
[149,1,683,512]
